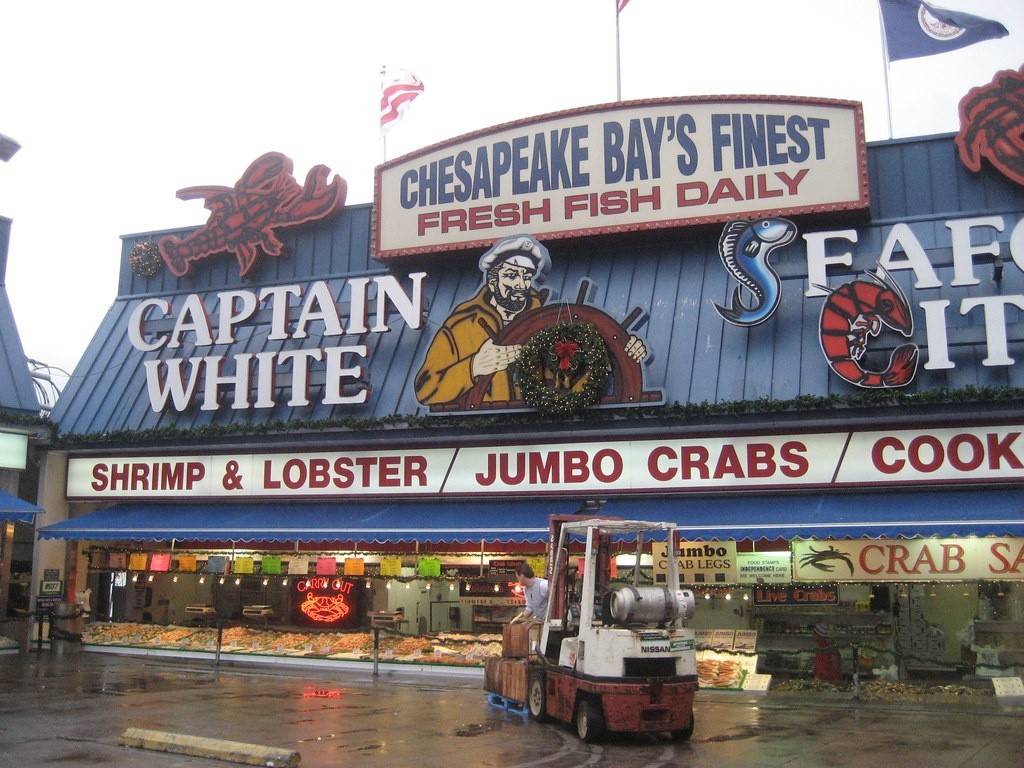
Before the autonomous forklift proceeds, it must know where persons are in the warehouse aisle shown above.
[515,564,549,624]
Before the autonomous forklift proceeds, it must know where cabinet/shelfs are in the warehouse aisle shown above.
[749,612,896,676]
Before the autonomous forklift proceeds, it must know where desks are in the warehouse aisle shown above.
[244,614,276,628]
[375,620,410,637]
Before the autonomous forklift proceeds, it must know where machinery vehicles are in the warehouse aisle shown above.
[528,514,699,741]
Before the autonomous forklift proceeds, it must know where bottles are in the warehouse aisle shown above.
[750,600,892,638]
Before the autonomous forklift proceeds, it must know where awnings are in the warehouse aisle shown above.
[35,488,1024,544]
[0,488,47,525]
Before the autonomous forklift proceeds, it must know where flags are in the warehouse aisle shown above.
[381,70,424,131]
[880,0,1010,64]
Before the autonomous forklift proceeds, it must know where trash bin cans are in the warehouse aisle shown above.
[50,602,85,655]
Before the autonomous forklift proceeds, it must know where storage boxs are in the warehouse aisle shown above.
[840,586,891,612]
[896,583,946,666]
[977,650,1016,676]
